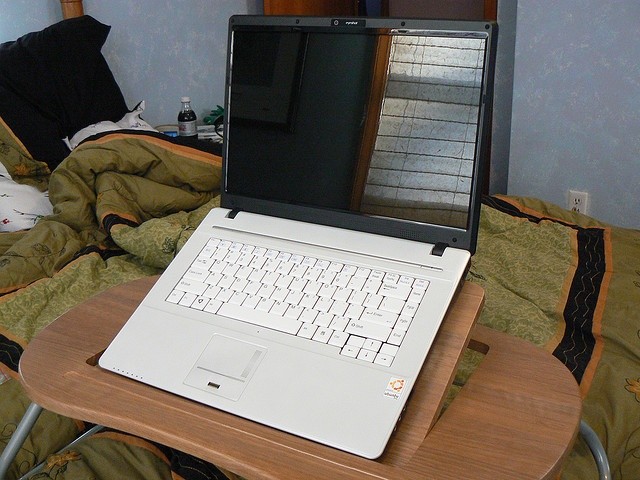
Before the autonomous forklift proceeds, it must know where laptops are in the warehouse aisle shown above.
[97,14,499,461]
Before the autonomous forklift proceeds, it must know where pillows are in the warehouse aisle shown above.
[0,16,129,191]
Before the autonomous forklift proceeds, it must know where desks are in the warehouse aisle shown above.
[1,274,611,480]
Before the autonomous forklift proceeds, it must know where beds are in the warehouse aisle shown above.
[1,0,636,480]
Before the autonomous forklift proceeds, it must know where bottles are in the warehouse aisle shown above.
[177,96,199,146]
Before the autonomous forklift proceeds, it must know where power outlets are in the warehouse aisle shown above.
[567,190,588,216]
